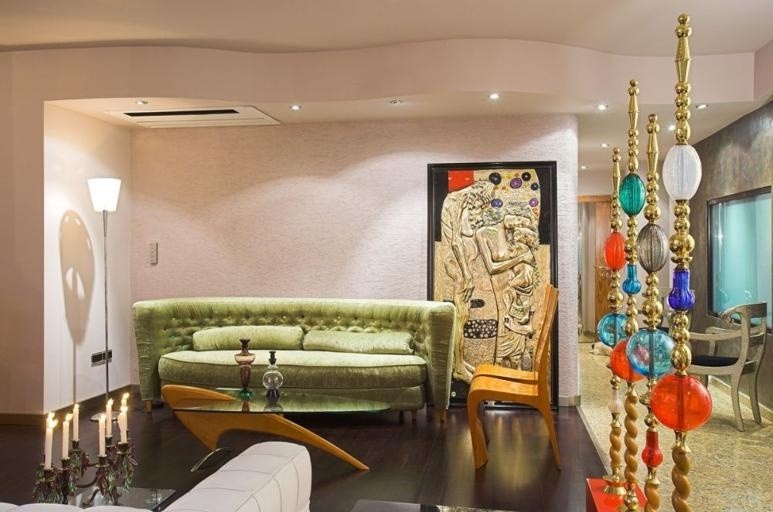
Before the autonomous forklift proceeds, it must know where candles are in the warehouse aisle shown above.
[44,392,131,468]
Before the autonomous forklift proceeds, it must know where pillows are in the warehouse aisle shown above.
[303,330,415,354]
[192,325,303,351]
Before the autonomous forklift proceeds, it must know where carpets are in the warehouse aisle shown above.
[349,499,518,512]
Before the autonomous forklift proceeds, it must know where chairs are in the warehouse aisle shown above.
[471,285,553,445]
[638,289,695,342]
[686,302,767,431]
[467,289,561,471]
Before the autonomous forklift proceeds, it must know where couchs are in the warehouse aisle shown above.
[132,296,455,424]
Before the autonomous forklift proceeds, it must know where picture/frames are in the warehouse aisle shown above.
[427,161,559,411]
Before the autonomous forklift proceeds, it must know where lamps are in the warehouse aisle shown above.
[87,178,122,422]
